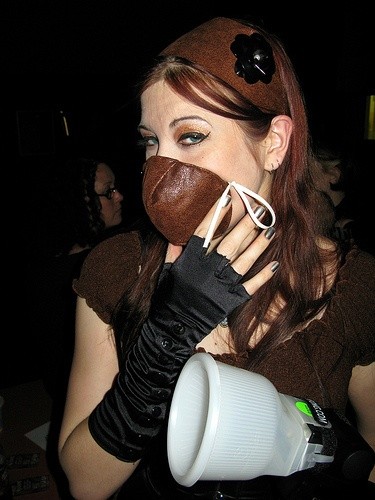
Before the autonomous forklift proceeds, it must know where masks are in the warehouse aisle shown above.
[141,156,277,247]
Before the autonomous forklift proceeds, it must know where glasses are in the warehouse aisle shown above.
[97,187,120,200]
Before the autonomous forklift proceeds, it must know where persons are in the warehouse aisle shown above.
[45,155,127,274]
[57,15,374,500]
